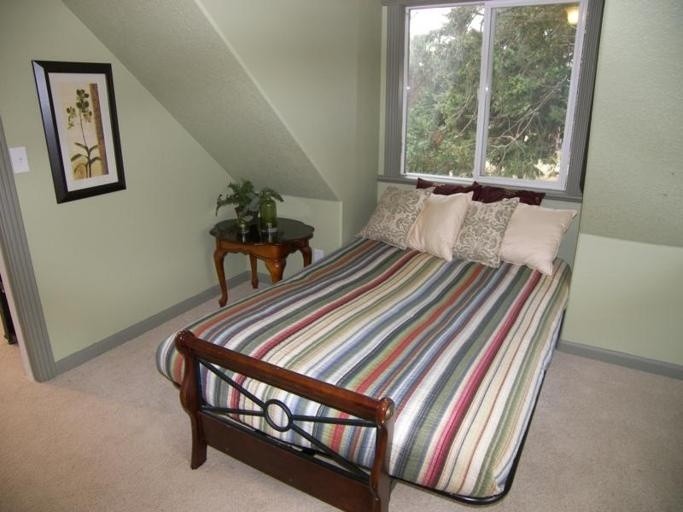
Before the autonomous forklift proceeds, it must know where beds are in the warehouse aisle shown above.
[155,171,576,512]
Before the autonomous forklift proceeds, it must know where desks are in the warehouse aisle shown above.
[208,219,316,307]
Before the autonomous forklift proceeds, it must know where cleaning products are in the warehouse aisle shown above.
[261,191,276,236]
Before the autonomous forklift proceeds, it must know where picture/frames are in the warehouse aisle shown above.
[29,53,126,206]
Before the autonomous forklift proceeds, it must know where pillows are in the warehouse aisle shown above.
[352,176,579,276]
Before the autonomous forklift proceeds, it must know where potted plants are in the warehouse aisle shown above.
[215,178,286,229]
